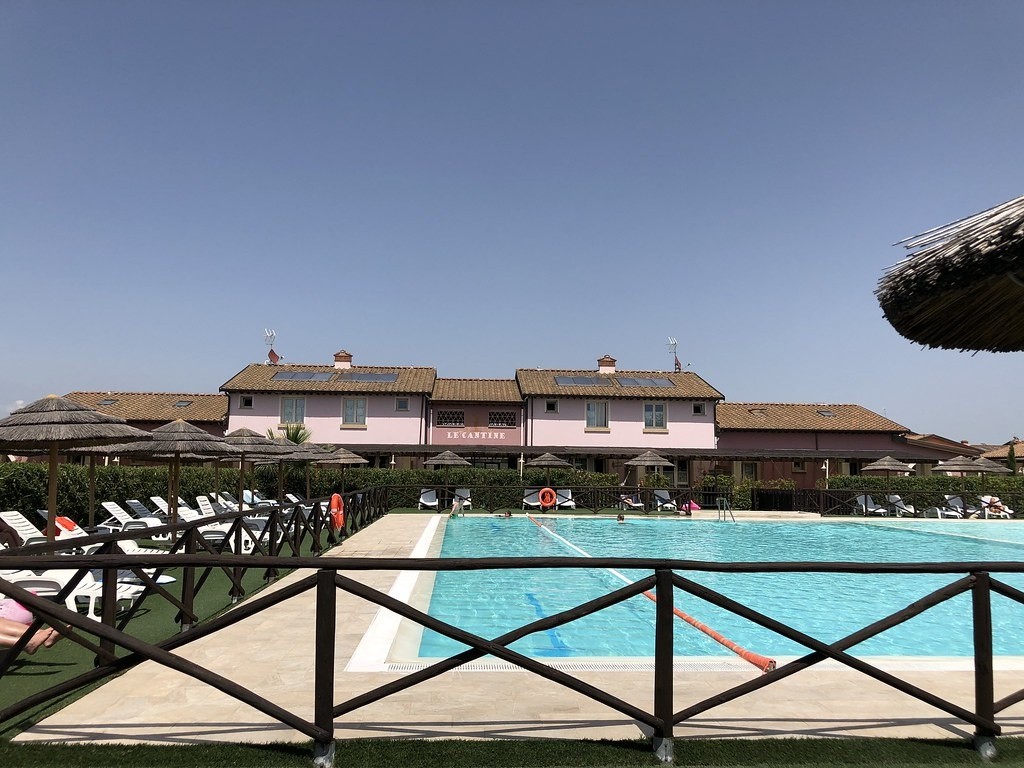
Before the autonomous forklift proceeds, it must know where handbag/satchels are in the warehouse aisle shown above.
[0,591,37,626]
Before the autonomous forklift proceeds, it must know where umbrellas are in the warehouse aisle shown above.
[624,450,676,487]
[316,448,370,492]
[931,455,994,490]
[860,456,916,489]
[422,450,473,485]
[973,455,1014,497]
[0,393,341,556]
[524,452,573,485]
[870,191,1024,359]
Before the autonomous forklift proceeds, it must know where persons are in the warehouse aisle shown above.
[0,617,73,655]
[499,510,512,519]
[639,480,647,510]
[983,498,1005,514]
[616,513,625,524]
[448,497,464,519]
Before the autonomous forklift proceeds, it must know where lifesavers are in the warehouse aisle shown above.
[538,488,557,507]
[330,492,344,528]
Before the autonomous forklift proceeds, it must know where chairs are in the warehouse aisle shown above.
[885,495,920,518]
[0,490,330,624]
[556,489,575,510]
[981,497,1010,519]
[418,489,439,511]
[620,495,644,511]
[522,489,542,510]
[856,495,887,516]
[944,495,982,519]
[452,489,472,510]
[654,490,677,512]
[920,507,962,519]
